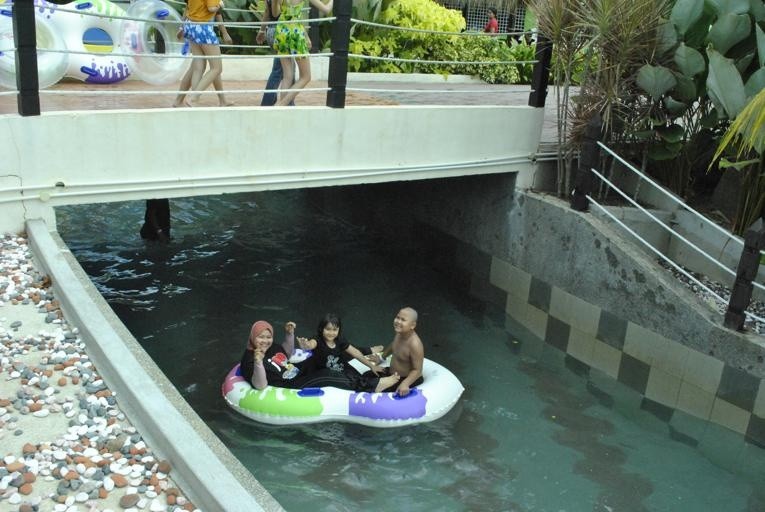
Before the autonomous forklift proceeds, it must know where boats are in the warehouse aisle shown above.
[0,0,143,89]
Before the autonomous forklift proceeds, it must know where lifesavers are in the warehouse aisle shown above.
[120,0,195,85]
[0,0,71,91]
[65,0,141,83]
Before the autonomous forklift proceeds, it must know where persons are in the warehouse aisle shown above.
[364,307,424,398]
[172,0,334,106]
[483,6,499,37]
[242,320,400,393]
[296,313,387,377]
[512,29,535,47]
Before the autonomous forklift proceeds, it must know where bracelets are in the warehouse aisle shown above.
[378,352,386,362]
